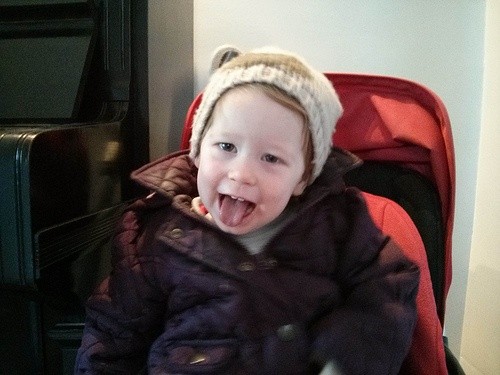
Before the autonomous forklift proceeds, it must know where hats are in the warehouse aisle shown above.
[189,53,343,186]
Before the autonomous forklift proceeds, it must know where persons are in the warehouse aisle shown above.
[75,46,421,375]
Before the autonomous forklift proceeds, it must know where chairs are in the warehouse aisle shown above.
[180,75,465,375]
